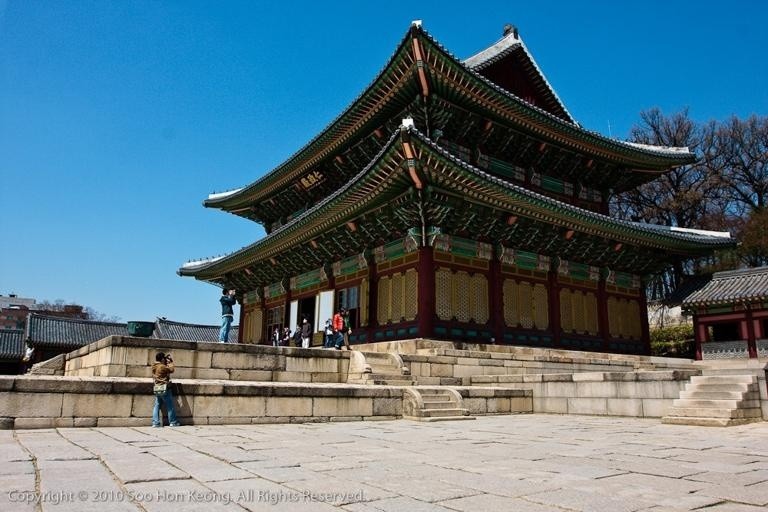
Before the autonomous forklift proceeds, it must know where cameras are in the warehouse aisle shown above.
[165,353,171,361]
[228,289,237,296]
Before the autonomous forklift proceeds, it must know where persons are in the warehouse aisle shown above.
[152,352,180,427]
[219,288,236,342]
[22,342,36,371]
[272,307,356,350]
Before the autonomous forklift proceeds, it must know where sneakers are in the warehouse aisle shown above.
[152,424,160,427]
[170,422,180,426]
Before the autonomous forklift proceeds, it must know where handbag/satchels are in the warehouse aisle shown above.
[153,384,168,397]
